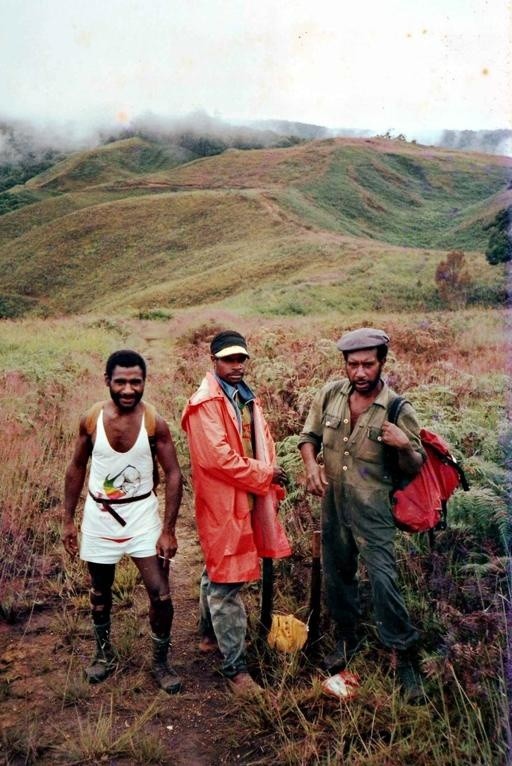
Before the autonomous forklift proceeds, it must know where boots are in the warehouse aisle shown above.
[146,630,183,694]
[85,622,120,684]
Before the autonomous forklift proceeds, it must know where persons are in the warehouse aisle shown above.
[180,330,289,697]
[296,327,428,688]
[60,349,183,692]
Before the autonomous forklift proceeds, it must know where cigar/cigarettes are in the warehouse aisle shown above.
[158,555,175,563]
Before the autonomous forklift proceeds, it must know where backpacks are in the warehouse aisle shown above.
[386,397,466,536]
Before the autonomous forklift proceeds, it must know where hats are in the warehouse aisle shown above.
[210,329,251,361]
[335,327,390,352]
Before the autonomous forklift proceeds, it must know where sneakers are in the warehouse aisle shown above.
[198,634,220,655]
[315,627,367,677]
[389,646,433,708]
[226,672,267,708]
